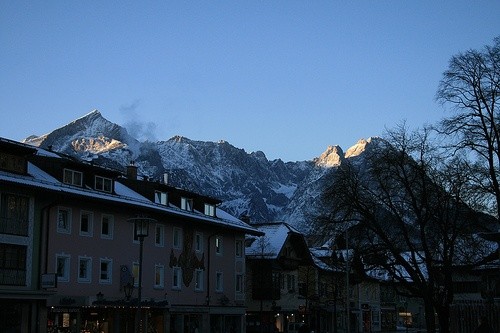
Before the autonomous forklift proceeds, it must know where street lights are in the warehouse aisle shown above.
[128,216,156,333]
